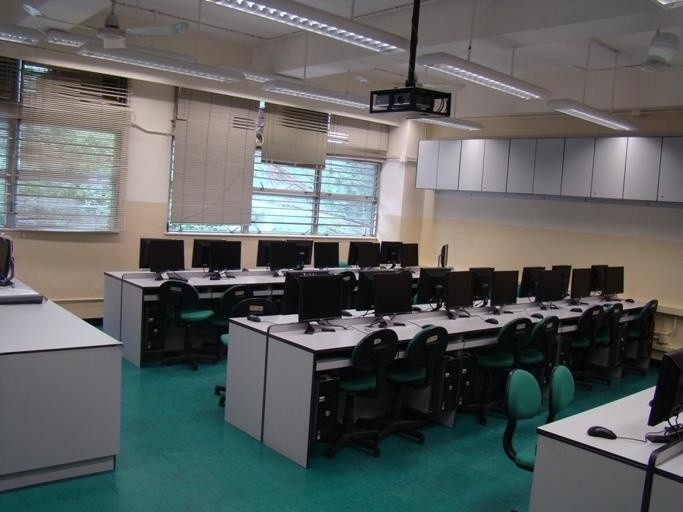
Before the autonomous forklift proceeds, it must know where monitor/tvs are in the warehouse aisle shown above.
[0,234,14,285]
[139,239,455,280]
[282,265,634,334]
[645,347,683,442]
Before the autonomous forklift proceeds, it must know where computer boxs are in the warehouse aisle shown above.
[142,301,166,353]
[558,333,575,366]
[429,353,461,414]
[616,325,629,363]
[309,371,340,443]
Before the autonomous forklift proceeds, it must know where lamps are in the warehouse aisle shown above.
[0,1,411,113]
[404,0,681,134]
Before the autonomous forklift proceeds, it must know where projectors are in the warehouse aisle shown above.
[369,86,452,119]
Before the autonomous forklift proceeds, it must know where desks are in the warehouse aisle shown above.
[524,382,683,512]
[0,273,124,492]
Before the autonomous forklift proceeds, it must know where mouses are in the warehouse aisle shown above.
[587,426,617,439]
[531,313,544,319]
[422,324,432,329]
[570,307,582,312]
[247,314,260,322]
[485,318,498,324]
[625,299,633,303]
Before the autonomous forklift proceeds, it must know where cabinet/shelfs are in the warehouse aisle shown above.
[562,136,628,201]
[623,137,683,204]
[506,137,564,199]
[415,138,461,190]
[459,139,509,195]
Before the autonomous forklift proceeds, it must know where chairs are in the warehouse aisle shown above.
[603,302,623,364]
[360,325,450,448]
[628,299,658,362]
[524,316,560,392]
[467,318,535,424]
[315,327,399,458]
[500,365,547,475]
[121,262,361,414]
[563,305,603,368]
